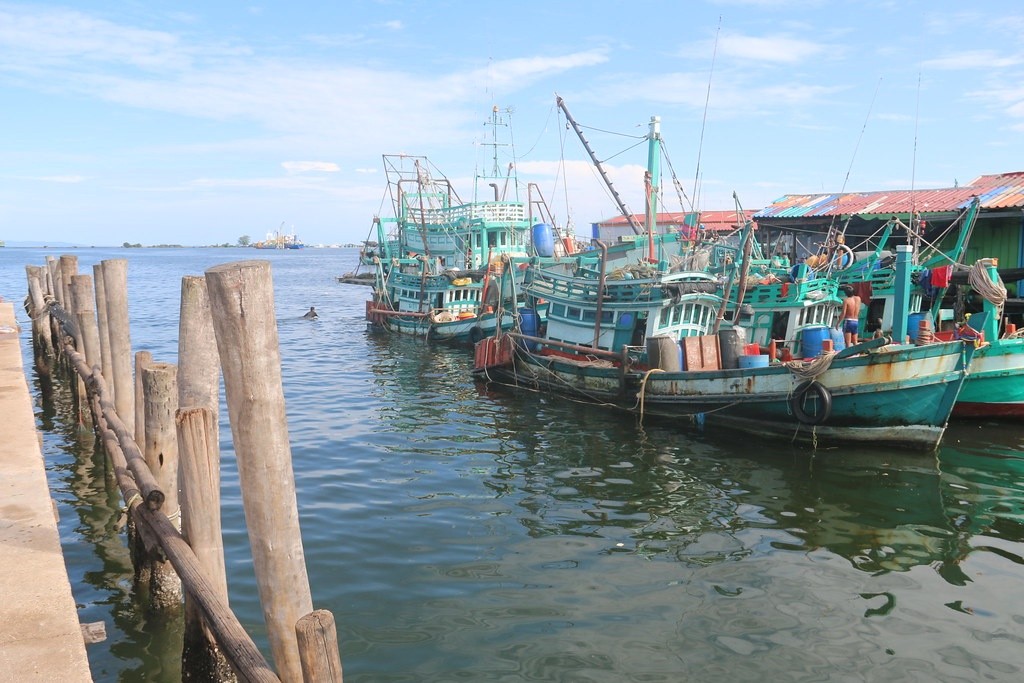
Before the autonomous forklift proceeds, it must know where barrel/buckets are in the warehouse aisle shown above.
[802,326,830,359]
[533,223,554,257]
[829,327,846,350]
[516,308,541,351]
[719,329,740,369]
[674,340,685,371]
[907,311,935,343]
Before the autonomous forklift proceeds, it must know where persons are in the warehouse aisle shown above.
[836,286,861,348]
[304,307,318,317]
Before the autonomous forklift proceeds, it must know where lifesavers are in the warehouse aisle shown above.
[791,381,832,426]
[826,243,854,270]
[469,326,485,349]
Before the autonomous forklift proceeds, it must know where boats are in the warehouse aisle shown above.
[336,81,1024,447]
